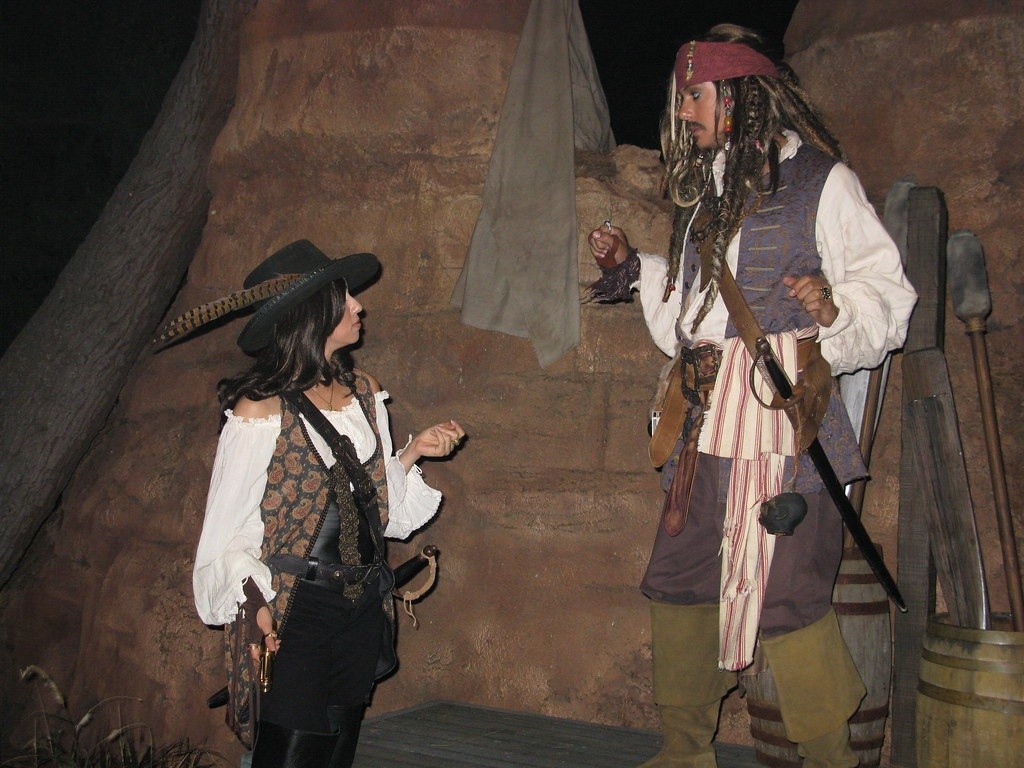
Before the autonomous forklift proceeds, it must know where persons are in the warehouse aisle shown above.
[588,23,918,768]
[192,239,465,768]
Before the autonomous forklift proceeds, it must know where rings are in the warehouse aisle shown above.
[604,221,611,233]
[452,440,460,446]
[264,630,278,640]
[821,287,831,300]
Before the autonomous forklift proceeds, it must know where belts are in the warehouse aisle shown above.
[648,333,822,467]
[269,553,381,585]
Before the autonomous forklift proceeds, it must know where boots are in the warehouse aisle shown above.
[632,599,737,768]
[757,606,865,768]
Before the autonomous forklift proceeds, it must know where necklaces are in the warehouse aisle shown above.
[311,381,333,411]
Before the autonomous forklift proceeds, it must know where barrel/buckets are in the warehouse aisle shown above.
[915,612,1024,768]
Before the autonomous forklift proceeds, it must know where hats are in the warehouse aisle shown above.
[148,237,381,354]
